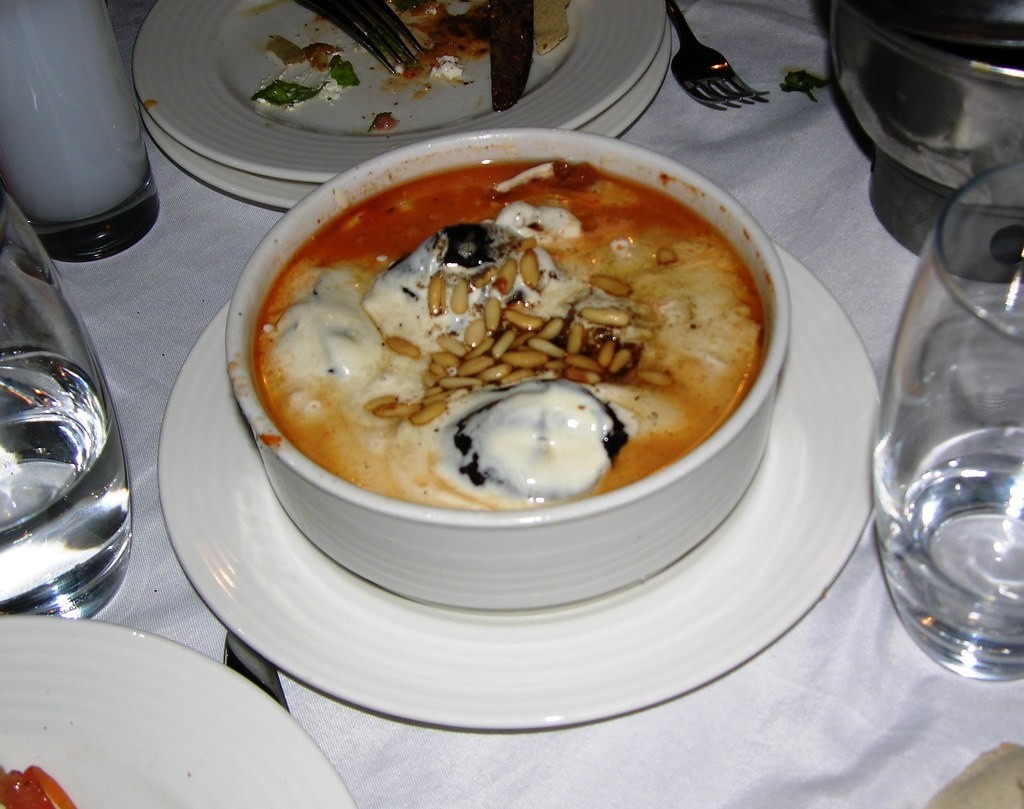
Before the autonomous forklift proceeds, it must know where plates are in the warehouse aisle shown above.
[133,0,666,185]
[137,16,671,210]
[0,614,358,808]
[157,245,882,731]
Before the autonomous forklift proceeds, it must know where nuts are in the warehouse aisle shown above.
[363,235,676,426]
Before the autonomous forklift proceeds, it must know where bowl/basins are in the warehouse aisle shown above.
[223,129,791,613]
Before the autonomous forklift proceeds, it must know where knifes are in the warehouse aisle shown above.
[489,0,536,111]
[223,630,287,711]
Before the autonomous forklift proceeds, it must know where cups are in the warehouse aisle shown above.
[0,183,134,615]
[871,159,1024,679]
[0,0,159,263]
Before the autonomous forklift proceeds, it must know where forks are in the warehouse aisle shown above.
[666,0,771,104]
[295,0,425,74]
